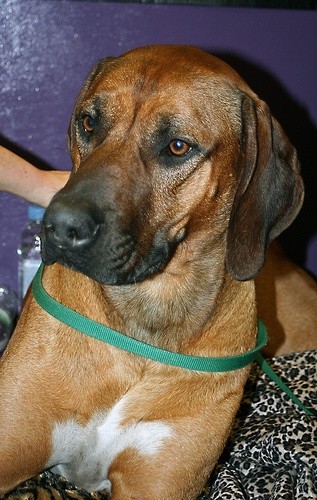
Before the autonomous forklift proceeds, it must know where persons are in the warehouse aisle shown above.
[0,143,72,208]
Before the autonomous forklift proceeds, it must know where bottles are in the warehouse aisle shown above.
[17,206,46,313]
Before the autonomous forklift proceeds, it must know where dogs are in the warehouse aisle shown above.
[0,43,317,500]
